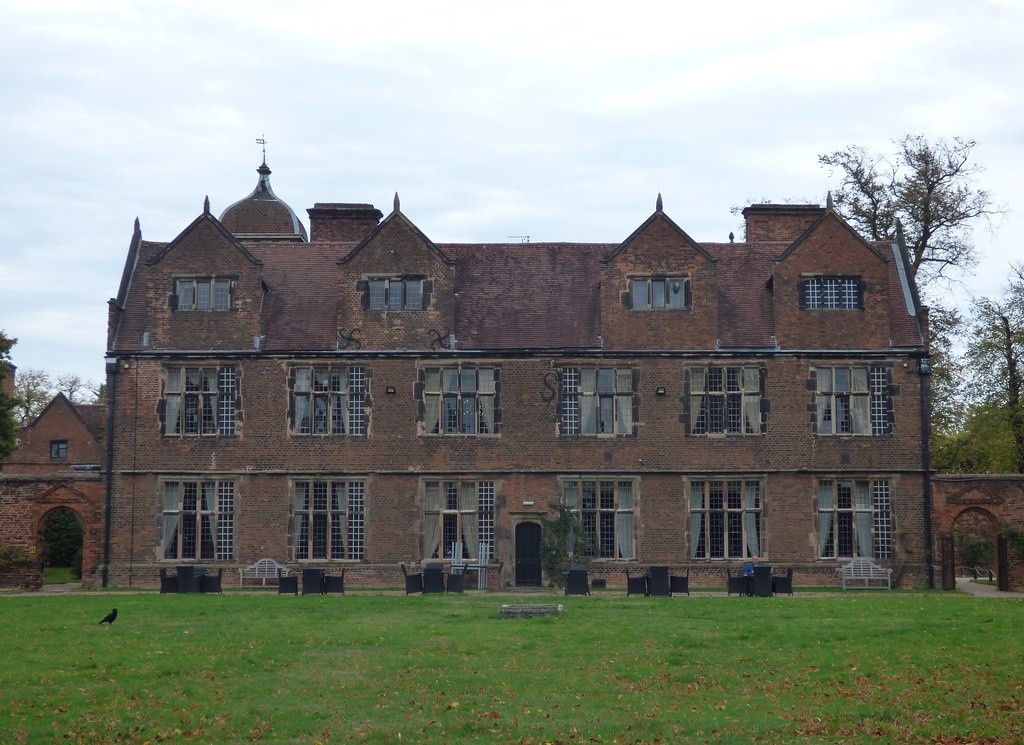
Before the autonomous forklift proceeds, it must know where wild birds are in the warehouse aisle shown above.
[99,608,118,625]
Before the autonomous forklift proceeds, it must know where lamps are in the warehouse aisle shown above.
[656,386,666,396]
[386,386,396,396]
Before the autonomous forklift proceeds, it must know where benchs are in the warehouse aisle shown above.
[239,559,290,590]
[835,557,893,592]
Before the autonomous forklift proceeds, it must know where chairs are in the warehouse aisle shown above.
[423,569,443,594]
[753,566,773,597]
[447,563,468,593]
[301,569,325,595]
[325,568,345,595]
[401,564,422,595]
[648,566,669,596]
[625,568,646,597]
[670,568,690,597]
[773,567,793,597]
[727,565,749,597]
[565,569,588,596]
[277,568,298,596]
[159,568,178,595]
[200,568,223,594]
[176,566,195,594]
[570,565,586,570]
